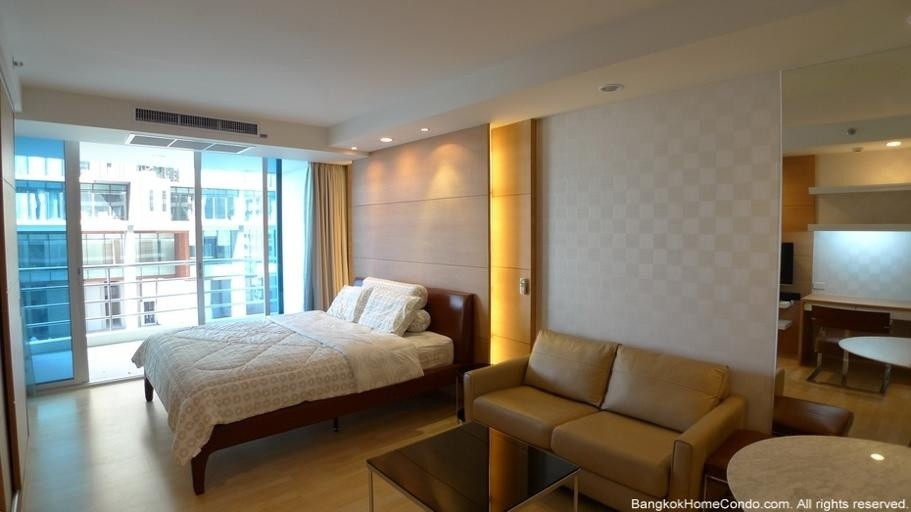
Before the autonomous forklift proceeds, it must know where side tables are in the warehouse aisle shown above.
[449,362,492,423]
[700,428,776,509]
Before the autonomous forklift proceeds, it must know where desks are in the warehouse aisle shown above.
[797,294,911,367]
[726,435,909,511]
[838,336,911,369]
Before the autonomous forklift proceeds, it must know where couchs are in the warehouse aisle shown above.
[463,328,747,512]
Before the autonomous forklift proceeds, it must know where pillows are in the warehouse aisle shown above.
[326,276,432,339]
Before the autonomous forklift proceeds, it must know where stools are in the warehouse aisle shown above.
[806,330,893,393]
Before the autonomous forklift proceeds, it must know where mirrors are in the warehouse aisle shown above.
[773,46,910,449]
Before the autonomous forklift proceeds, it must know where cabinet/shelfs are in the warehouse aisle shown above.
[805,183,911,232]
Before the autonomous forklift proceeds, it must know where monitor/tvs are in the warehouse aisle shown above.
[780,242,794,286]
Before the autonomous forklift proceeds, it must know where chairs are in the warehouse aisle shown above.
[774,395,854,437]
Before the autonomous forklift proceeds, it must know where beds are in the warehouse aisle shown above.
[143,275,474,496]
